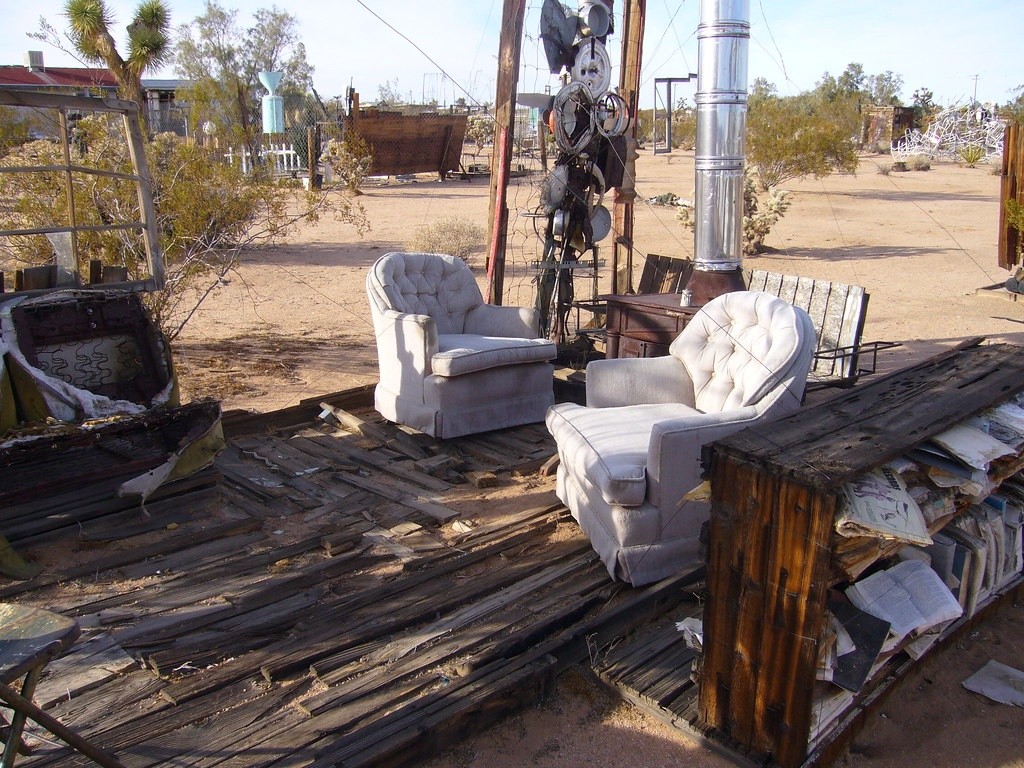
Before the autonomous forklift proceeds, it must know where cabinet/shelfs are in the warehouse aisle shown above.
[695,336,1024,768]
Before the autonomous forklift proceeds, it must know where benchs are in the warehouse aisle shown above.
[574,255,902,405]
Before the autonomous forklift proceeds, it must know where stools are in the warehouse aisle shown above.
[0,602,127,768]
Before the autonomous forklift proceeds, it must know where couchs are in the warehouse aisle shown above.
[366,252,558,438]
[546,290,815,586]
